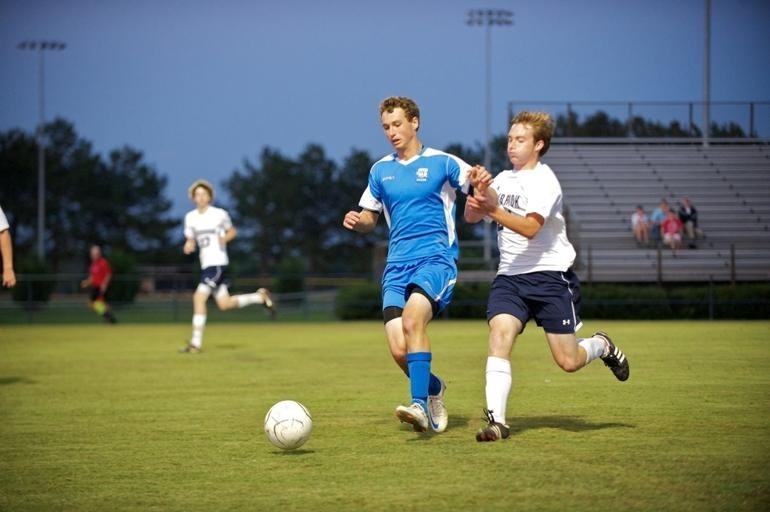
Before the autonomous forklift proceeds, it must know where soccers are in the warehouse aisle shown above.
[264,400,313,450]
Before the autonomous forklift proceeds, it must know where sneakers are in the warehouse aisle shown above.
[476,422,511,442]
[427,375,449,433]
[592,330,629,381]
[256,287,277,321]
[396,403,428,434]
[178,340,207,354]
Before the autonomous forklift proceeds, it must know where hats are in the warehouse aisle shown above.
[188,180,215,200]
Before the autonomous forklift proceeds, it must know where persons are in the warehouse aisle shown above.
[629,197,707,253]
[342,96,490,433]
[0,208,17,292]
[464,110,630,444]
[179,179,275,353]
[79,245,118,328]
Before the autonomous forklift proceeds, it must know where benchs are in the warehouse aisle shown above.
[535,136,769,285]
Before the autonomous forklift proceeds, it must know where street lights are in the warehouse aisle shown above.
[16,40,67,259]
[467,6,516,259]
